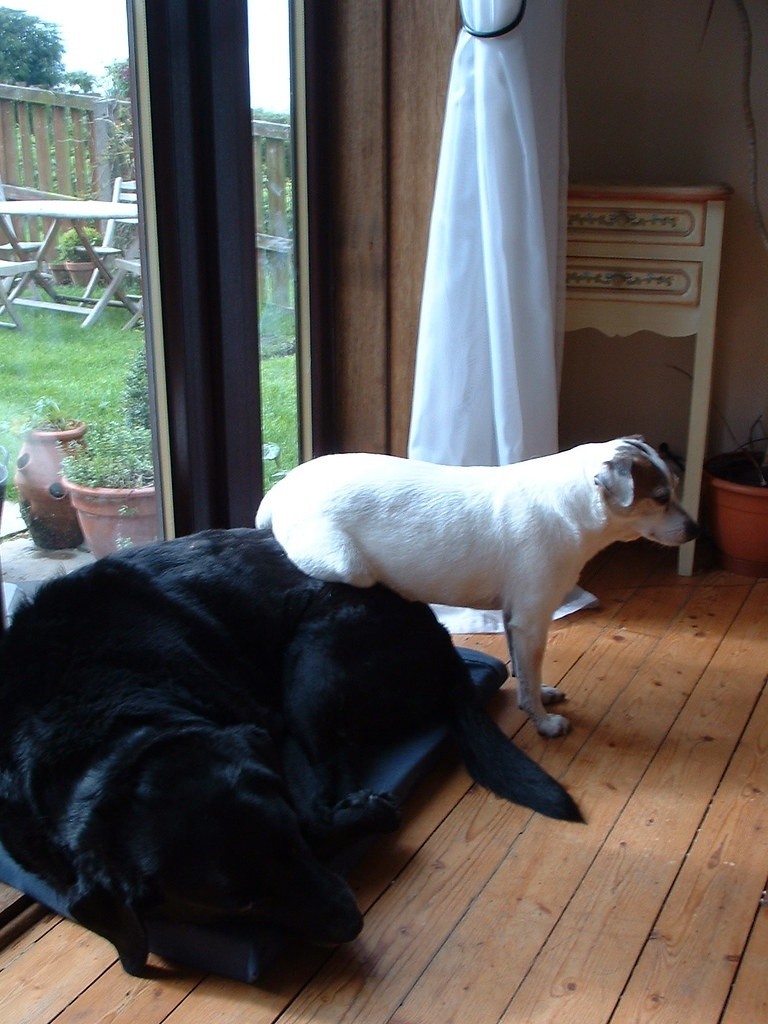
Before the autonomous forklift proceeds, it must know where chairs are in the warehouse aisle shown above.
[0,241,43,305]
[78,176,142,307]
[0,258,39,330]
[79,259,143,332]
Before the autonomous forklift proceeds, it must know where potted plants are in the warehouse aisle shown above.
[56,342,159,561]
[9,397,91,550]
[47,244,71,285]
[59,225,104,289]
[664,363,768,579]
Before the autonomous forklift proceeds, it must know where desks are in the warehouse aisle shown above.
[562,177,734,577]
[0,200,138,329]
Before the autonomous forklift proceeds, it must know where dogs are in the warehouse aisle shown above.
[256,436,700,738]
[0,526,585,987]
